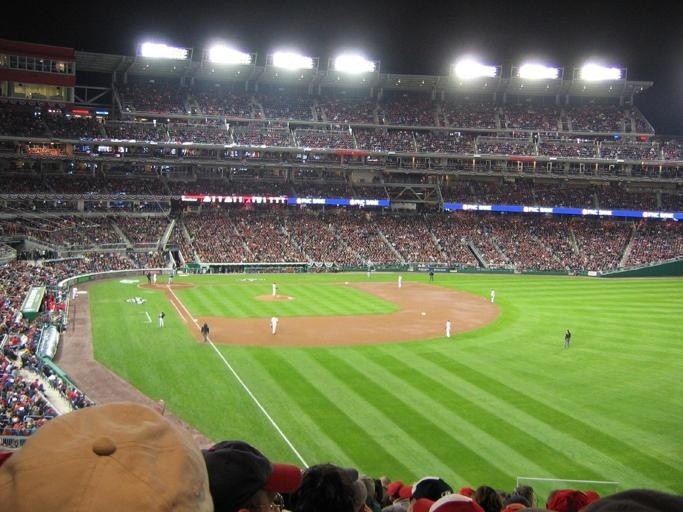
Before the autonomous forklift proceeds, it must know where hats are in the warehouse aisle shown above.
[301,462,601,512]
[1,400,217,512]
[196,439,305,512]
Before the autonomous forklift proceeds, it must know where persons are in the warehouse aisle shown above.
[1,50,681,271]
[428,272,433,281]
[200,322,209,343]
[146,271,151,285]
[272,282,276,297]
[489,288,495,304]
[562,329,571,350]
[0,394,683,511]
[269,315,278,335]
[158,311,164,328]
[445,319,451,337]
[0,270,110,394]
[168,273,173,285]
[397,275,403,289]
[152,273,157,285]
